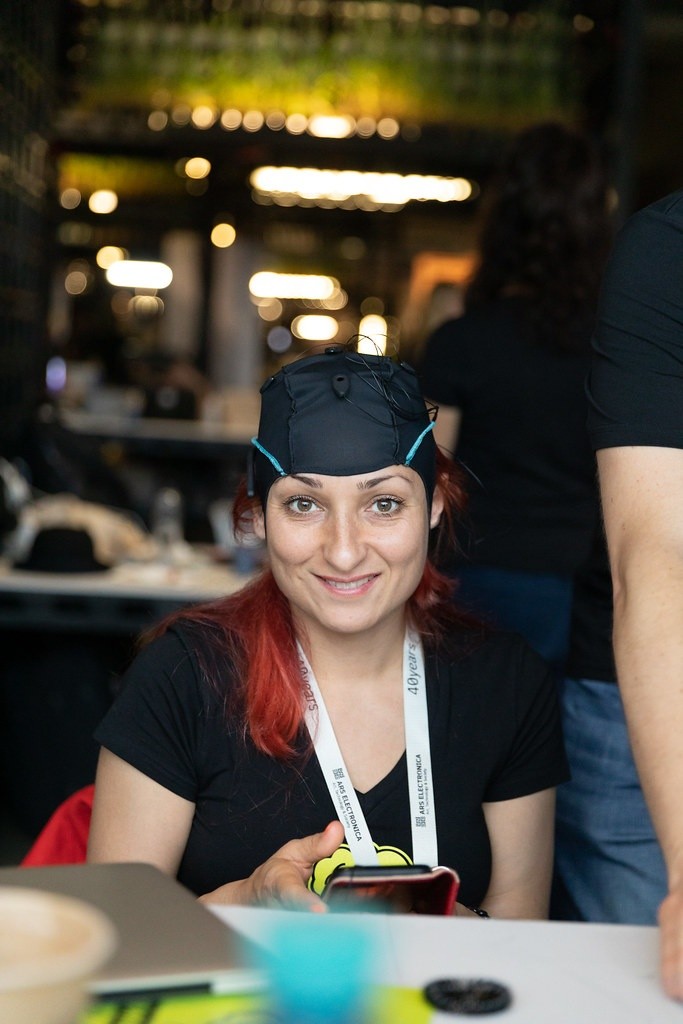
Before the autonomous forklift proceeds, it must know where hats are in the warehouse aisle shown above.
[243,333,439,521]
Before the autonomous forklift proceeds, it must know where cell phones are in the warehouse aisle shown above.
[320,864,433,899]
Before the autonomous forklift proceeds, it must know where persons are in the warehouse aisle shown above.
[87,349,573,920]
[416,185,683,1003]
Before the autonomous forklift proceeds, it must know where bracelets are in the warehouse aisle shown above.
[470,908,489,919]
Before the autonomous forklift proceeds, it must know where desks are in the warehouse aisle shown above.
[0,542,261,867]
[211,904,683,1024]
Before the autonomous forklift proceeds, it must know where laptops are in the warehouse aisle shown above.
[0,862,276,1004]
